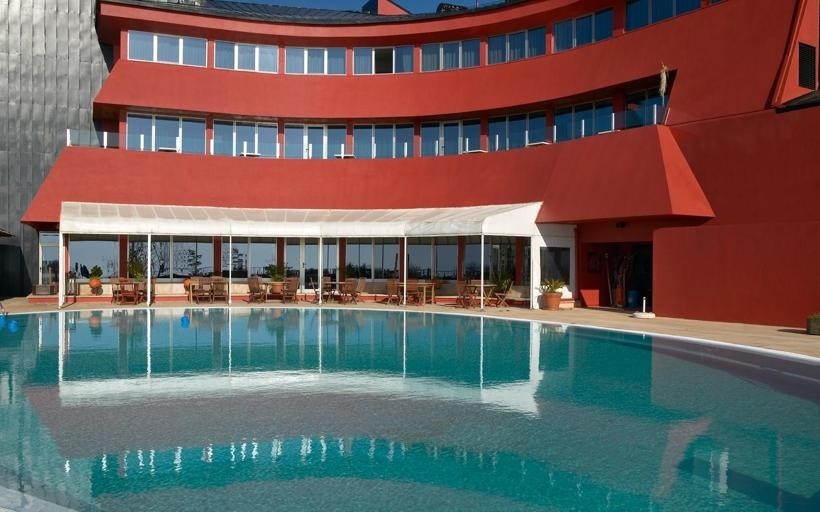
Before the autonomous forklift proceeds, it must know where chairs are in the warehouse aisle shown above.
[110,277,156,305]
[310,276,366,304]
[184,276,229,305]
[455,278,513,311]
[87,307,434,334]
[246,276,299,304]
[385,279,441,306]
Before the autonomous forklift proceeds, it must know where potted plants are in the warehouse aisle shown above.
[534,278,568,311]
[440,272,453,297]
[266,263,292,294]
[806,311,820,335]
[83,265,103,288]
